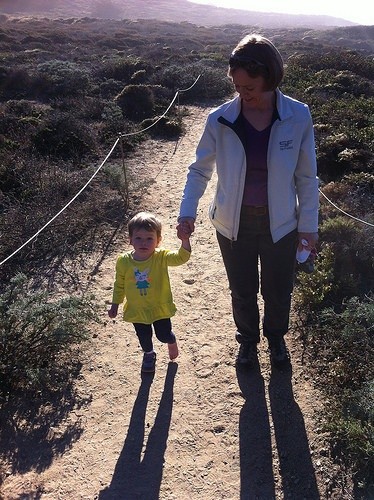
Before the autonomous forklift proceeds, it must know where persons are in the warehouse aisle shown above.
[108,211,192,373]
[176,34,319,370]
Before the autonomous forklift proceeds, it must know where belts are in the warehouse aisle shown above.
[241,205,268,216]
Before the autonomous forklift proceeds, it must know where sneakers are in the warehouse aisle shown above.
[268,336,291,368]
[141,352,157,373]
[235,343,260,369]
